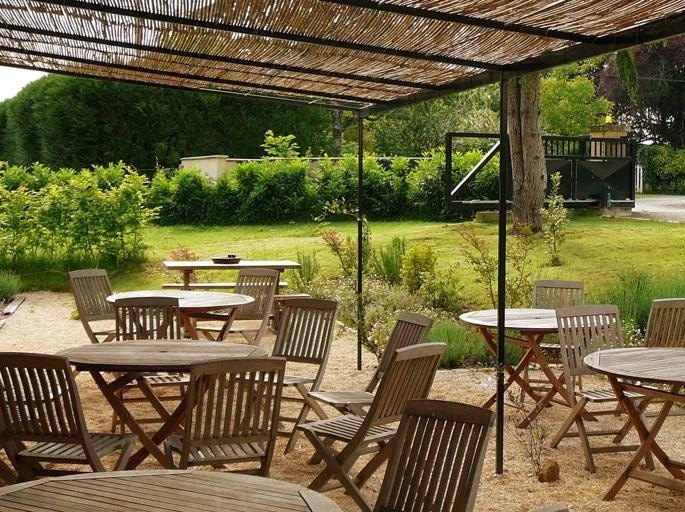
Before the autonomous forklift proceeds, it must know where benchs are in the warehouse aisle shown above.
[249,294,312,301]
[161,282,289,290]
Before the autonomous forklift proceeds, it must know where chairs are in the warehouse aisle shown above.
[68,268,167,382]
[235,298,342,456]
[371,399,495,512]
[0,400,42,482]
[0,352,139,477]
[110,296,192,434]
[0,377,71,404]
[293,342,448,511]
[164,355,286,476]
[519,280,584,404]
[195,267,279,392]
[550,304,655,471]
[615,298,685,418]
[306,310,433,469]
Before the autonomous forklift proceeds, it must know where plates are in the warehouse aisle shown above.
[211,257,243,264]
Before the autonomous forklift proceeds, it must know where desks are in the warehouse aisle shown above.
[105,289,255,341]
[582,348,685,501]
[162,260,302,334]
[458,309,617,422]
[0,470,347,512]
[56,339,269,469]
